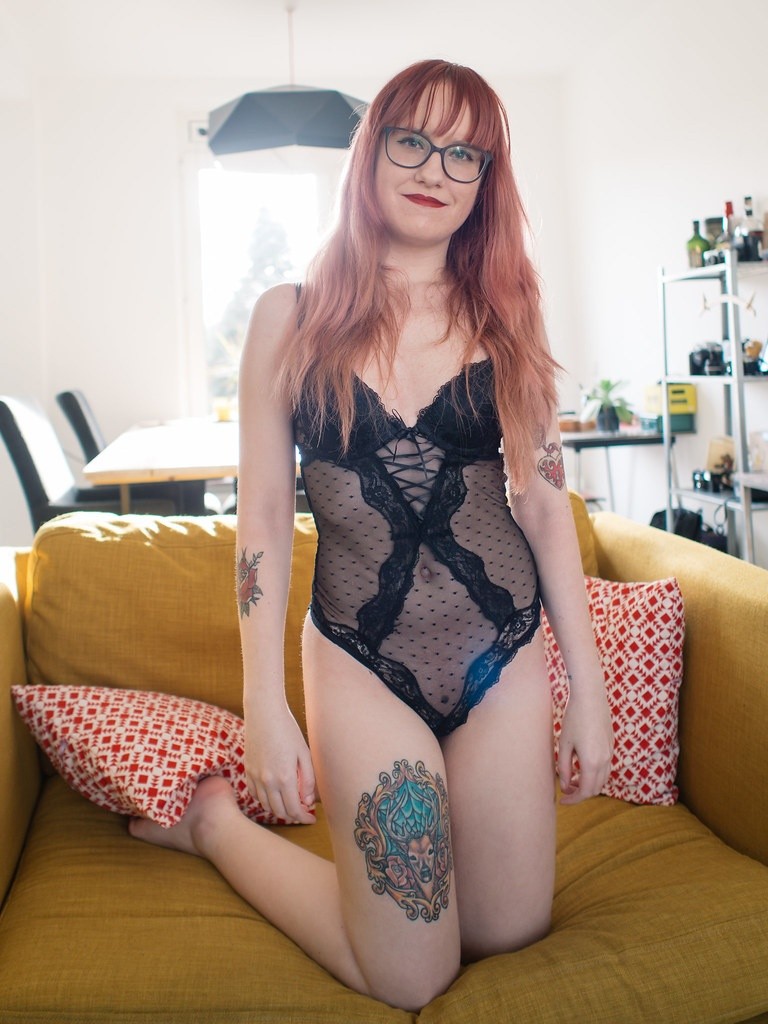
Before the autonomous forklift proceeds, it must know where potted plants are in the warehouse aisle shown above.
[581,380,636,433]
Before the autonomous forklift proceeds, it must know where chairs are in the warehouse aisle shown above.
[56,386,206,517]
[1,402,179,536]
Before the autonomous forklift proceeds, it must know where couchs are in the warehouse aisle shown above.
[0,494,767,1023]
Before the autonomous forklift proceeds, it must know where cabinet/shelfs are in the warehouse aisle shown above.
[661,248,768,574]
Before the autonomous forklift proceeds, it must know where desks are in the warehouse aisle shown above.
[81,423,314,514]
[559,432,677,520]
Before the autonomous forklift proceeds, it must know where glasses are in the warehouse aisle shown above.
[383,126,494,183]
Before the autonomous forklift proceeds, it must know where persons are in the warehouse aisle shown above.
[128,58,614,1014]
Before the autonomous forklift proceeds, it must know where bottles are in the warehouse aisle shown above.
[736,196,764,261]
[714,202,744,263]
[687,221,710,267]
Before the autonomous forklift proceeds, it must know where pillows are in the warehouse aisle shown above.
[11,686,319,825]
[530,574,685,808]
